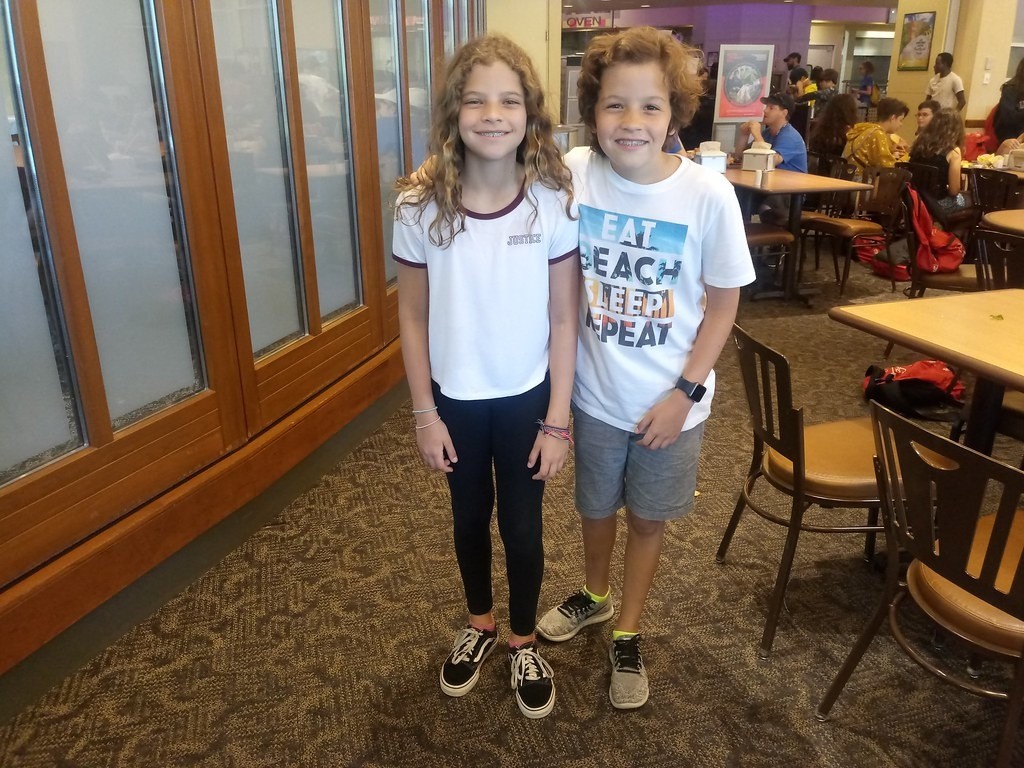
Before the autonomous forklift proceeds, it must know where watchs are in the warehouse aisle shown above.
[676,376,706,403]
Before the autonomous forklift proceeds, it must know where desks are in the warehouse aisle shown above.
[982,209,1024,234]
[828,286,1024,575]
[946,157,1024,209]
[720,163,872,304]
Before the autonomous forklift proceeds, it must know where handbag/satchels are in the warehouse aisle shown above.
[876,237,910,264]
[869,254,912,282]
[964,132,991,161]
[855,234,886,268]
[904,182,966,273]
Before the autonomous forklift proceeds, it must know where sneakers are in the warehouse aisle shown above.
[607,633,651,709]
[439,628,500,696]
[506,638,556,719]
[537,586,614,640]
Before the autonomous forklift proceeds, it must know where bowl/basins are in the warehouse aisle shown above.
[974,165,983,167]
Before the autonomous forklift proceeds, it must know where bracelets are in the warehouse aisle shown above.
[536,418,575,445]
[416,417,440,428]
[412,406,437,413]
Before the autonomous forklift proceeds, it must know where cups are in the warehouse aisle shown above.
[991,155,1009,168]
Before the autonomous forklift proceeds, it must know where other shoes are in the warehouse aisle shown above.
[766,246,785,267]
[961,254,989,264]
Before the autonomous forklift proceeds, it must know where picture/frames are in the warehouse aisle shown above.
[897,10,936,72]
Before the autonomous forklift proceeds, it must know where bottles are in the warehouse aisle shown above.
[893,152,899,160]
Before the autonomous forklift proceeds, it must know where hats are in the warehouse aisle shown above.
[759,89,796,115]
[785,53,801,62]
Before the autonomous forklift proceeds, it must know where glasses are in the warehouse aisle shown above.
[914,112,933,118]
[774,92,788,105]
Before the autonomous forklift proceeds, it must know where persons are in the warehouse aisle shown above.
[392,35,578,720]
[926,52,966,112]
[852,61,874,108]
[734,92,808,266]
[410,27,756,709]
[663,62,734,165]
[983,57,1024,153]
[842,97,911,212]
[296,57,430,166]
[910,107,981,221]
[810,94,857,155]
[782,52,840,118]
[917,100,941,134]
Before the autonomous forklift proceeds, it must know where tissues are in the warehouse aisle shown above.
[694,141,727,173]
[741,142,776,172]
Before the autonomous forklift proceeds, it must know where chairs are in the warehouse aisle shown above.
[546,144,1024,767]
